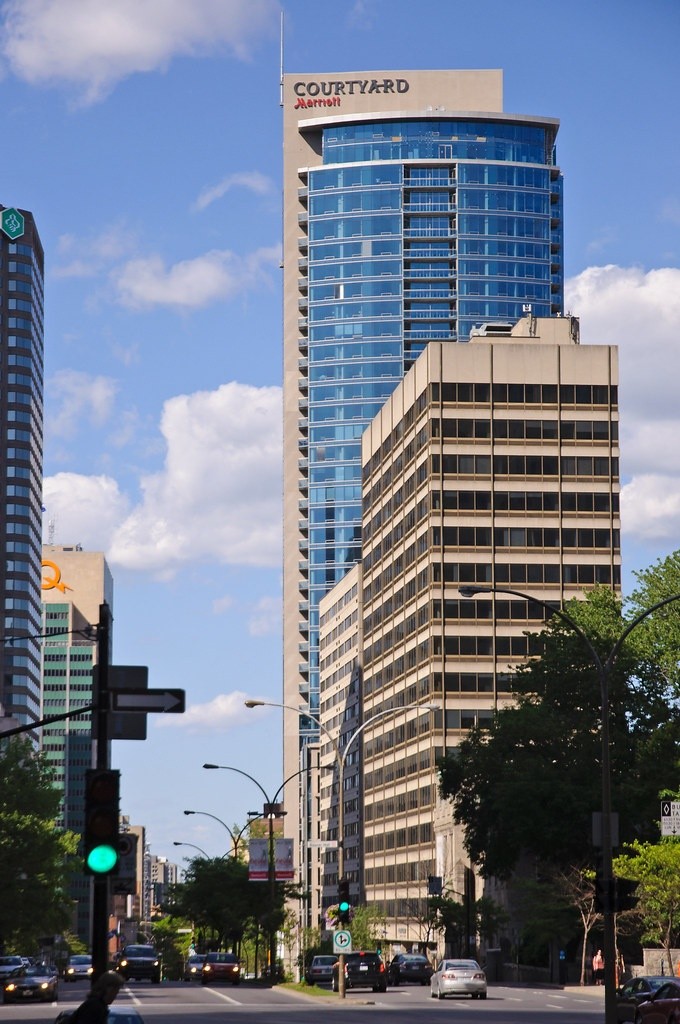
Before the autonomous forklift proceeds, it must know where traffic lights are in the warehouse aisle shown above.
[614,875,641,914]
[339,880,350,922]
[84,769,120,876]
[377,942,381,955]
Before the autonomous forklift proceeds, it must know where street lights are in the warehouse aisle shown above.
[203,763,338,979]
[457,583,680,1023]
[183,809,287,859]
[245,698,442,996]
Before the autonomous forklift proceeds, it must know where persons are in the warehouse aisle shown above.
[615,948,625,989]
[61,970,125,1024]
[593,949,605,986]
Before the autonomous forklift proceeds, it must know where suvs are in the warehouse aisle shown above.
[116,945,165,984]
[304,955,338,986]
[330,950,387,993]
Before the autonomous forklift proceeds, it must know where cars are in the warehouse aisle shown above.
[387,953,433,986]
[3,965,60,1003]
[615,975,677,1024]
[184,955,207,981]
[201,952,244,986]
[0,955,31,980]
[64,954,93,982]
[634,980,680,1024]
[429,959,487,999]
